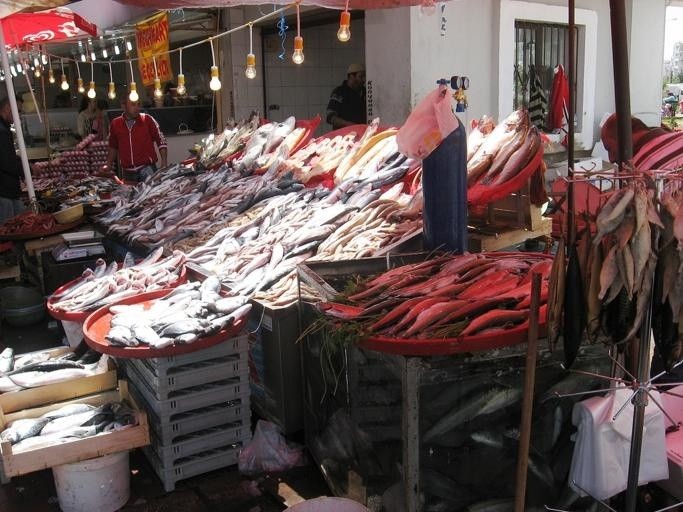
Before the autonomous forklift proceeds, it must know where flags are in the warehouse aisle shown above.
[135,10,174,86]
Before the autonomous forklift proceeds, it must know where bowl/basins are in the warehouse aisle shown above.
[0,287,44,325]
[52,203,83,224]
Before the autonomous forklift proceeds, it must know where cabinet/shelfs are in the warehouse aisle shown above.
[116,335,253,492]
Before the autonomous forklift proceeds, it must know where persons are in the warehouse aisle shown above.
[662,91,676,115]
[72,94,111,140]
[325,63,366,131]
[98,89,168,182]
[600,112,683,394]
[0,94,25,218]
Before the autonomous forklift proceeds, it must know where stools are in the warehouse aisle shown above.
[0,265,21,286]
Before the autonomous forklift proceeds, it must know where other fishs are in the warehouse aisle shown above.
[47,108,554,351]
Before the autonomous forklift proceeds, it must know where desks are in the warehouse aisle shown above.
[40,251,114,296]
[24,223,97,296]
[467,216,552,252]
[297,252,622,512]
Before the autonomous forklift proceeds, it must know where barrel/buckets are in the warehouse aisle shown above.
[52,453,130,511]
[282,497,371,512]
